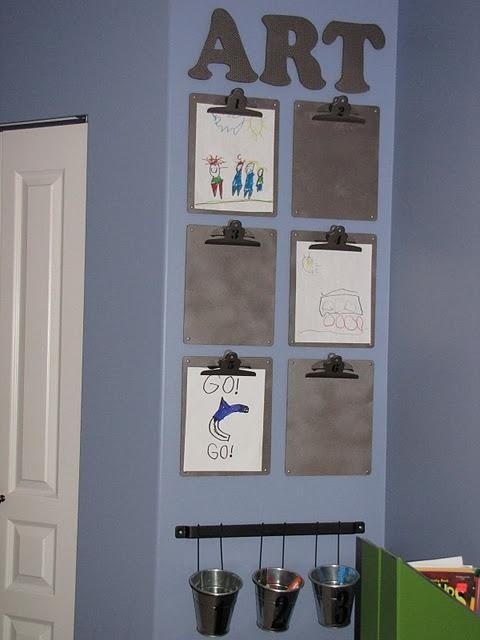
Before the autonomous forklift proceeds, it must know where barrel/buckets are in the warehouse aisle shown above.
[189,568,243,637]
[252,567,304,632]
[308,565,360,627]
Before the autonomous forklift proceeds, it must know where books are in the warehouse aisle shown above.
[416,563,480,616]
